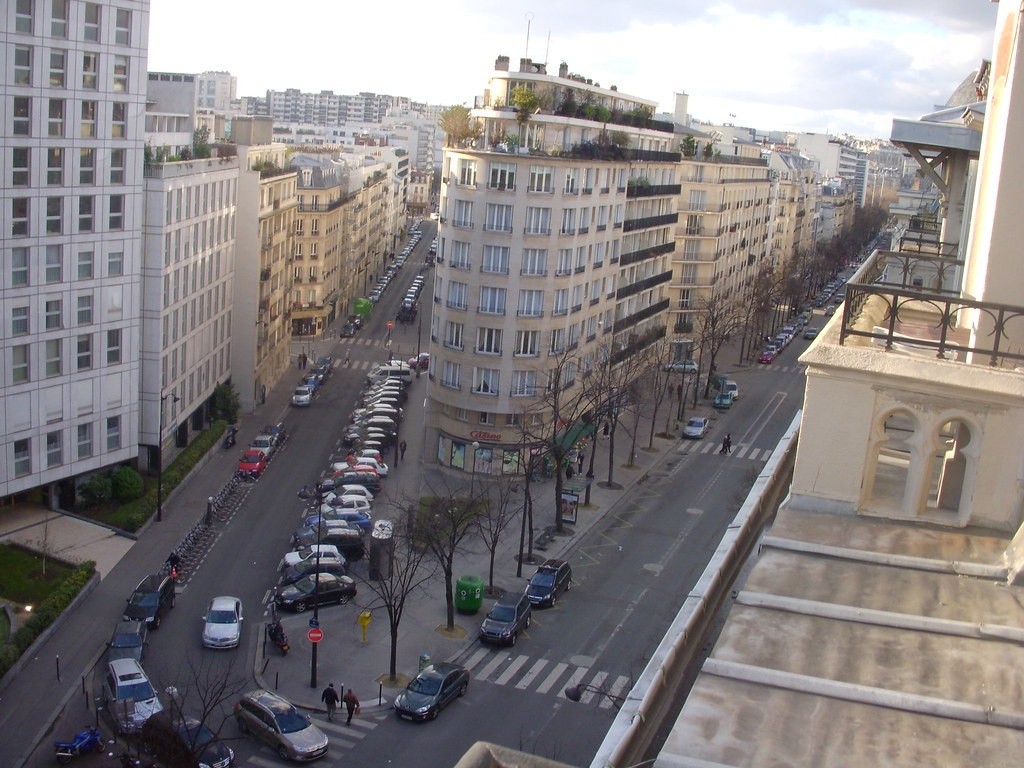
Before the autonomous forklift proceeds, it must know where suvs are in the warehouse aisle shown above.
[122,573,176,631]
[480,593,533,647]
[102,658,164,737]
[141,706,239,768]
[234,689,333,765]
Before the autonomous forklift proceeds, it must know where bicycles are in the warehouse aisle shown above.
[158,561,187,585]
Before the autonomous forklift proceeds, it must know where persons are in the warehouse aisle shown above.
[400,440,407,459]
[603,422,610,440]
[725,332,730,344]
[677,384,682,400]
[668,383,674,399]
[322,683,339,721]
[272,620,284,638]
[577,452,584,475]
[344,349,350,364]
[165,553,180,573]
[720,434,732,453]
[343,688,359,725]
[566,464,574,480]
[298,353,307,370]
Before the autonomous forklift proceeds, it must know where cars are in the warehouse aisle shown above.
[713,374,741,410]
[524,557,573,611]
[682,415,710,439]
[663,358,699,374]
[758,227,890,365]
[233,214,437,614]
[104,618,149,670]
[393,661,471,724]
[201,595,244,650]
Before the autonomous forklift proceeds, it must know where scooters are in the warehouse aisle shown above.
[267,616,292,655]
[224,425,239,449]
[107,737,142,768]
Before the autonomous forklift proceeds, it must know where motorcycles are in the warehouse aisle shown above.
[52,724,106,766]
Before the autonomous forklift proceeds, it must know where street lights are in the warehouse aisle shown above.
[414,301,424,378]
[510,480,529,578]
[295,481,323,688]
[156,389,182,523]
[384,356,404,467]
[469,440,480,498]
[165,686,179,768]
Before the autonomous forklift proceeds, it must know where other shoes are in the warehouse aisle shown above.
[346,722,351,726]
[580,473,583,476]
[725,453,727,455]
[729,451,731,453]
[328,717,331,721]
[719,451,721,454]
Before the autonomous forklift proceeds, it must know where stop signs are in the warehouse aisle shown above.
[386,321,394,328]
[307,627,323,643]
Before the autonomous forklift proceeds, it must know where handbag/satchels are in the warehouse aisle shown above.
[355,706,360,715]
